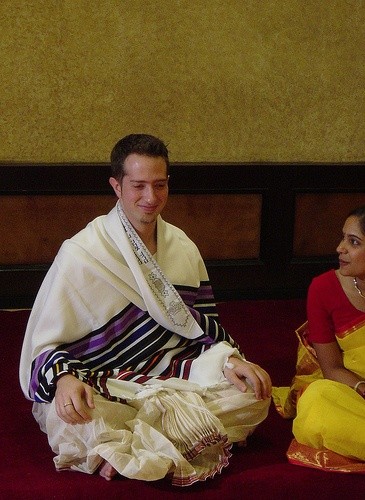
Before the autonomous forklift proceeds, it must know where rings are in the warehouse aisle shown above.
[63,403,73,409]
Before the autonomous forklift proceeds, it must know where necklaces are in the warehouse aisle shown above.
[353,276,364,298]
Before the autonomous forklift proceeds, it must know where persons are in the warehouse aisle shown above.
[19,133,274,486]
[273,208,365,470]
